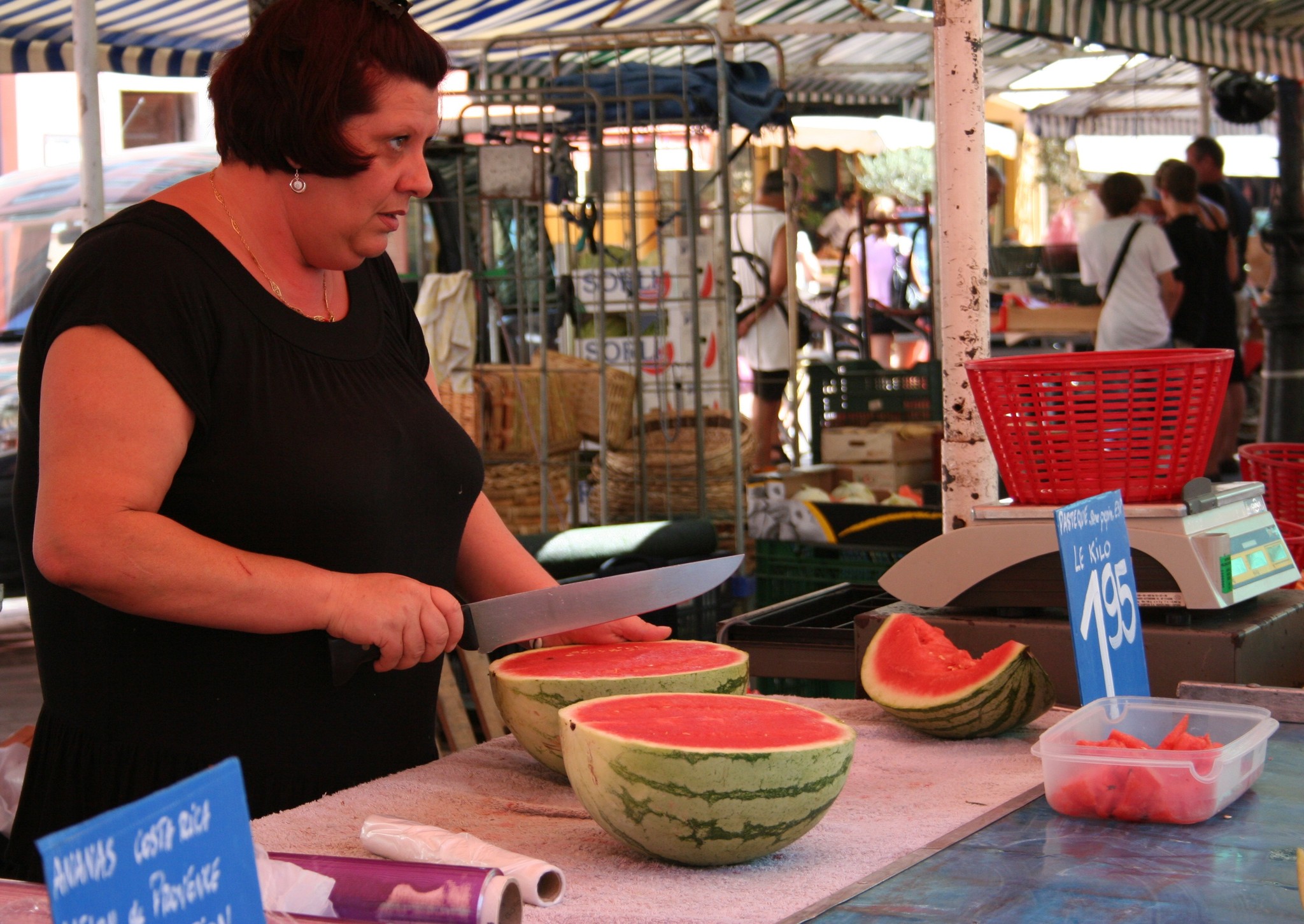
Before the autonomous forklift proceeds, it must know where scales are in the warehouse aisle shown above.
[878,477,1301,618]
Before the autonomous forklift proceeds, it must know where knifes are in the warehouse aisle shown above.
[371,553,747,661]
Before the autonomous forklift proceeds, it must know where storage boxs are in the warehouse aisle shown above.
[807,358,941,464]
[756,502,942,700]
[572,235,729,412]
[748,465,832,497]
[832,462,932,487]
[821,425,933,462]
[1030,695,1279,824]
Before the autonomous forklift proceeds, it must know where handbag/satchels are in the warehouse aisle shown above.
[786,310,812,347]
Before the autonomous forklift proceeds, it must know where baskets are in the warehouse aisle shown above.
[1238,443,1304,526]
[1274,519,1304,590]
[964,347,1235,507]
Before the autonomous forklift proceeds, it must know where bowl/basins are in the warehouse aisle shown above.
[1030,696,1281,824]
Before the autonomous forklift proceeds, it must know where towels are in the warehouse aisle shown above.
[410,269,476,395]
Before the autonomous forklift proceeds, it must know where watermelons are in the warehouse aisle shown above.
[487,638,751,777]
[860,611,1056,740]
[1056,707,1255,822]
[580,315,669,338]
[576,244,635,269]
[558,693,857,864]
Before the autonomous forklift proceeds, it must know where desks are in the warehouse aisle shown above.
[251,692,1304,924]
[991,306,1099,339]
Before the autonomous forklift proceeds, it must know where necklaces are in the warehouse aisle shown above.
[209,166,336,322]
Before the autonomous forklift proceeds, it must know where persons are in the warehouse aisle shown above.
[728,135,1262,475]
[6,0,672,885]
